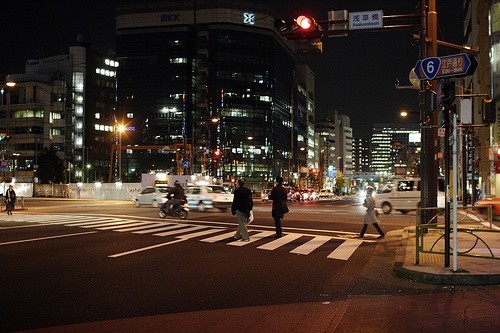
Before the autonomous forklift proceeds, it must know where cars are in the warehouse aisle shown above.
[260,184,377,204]
[132,185,174,209]
[153,179,234,214]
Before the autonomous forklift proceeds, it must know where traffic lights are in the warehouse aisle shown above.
[0,134,10,141]
[294,13,318,33]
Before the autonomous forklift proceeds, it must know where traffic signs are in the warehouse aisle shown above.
[412,52,478,81]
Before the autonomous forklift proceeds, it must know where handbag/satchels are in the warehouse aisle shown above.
[247,209,253,224]
[272,202,289,216]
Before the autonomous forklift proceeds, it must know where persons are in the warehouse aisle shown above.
[356,186,385,239]
[476,186,481,200]
[5,185,16,215]
[163,180,185,212]
[230,179,254,242]
[267,176,289,237]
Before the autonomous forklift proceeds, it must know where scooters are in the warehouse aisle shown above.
[158,195,191,220]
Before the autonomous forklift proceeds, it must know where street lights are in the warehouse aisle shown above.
[0,80,16,161]
[117,124,126,183]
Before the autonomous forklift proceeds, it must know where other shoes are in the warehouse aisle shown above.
[234,233,238,239]
[241,238,250,241]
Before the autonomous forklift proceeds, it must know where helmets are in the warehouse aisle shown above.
[173,179,179,185]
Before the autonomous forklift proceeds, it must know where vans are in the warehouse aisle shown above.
[373,175,445,214]
